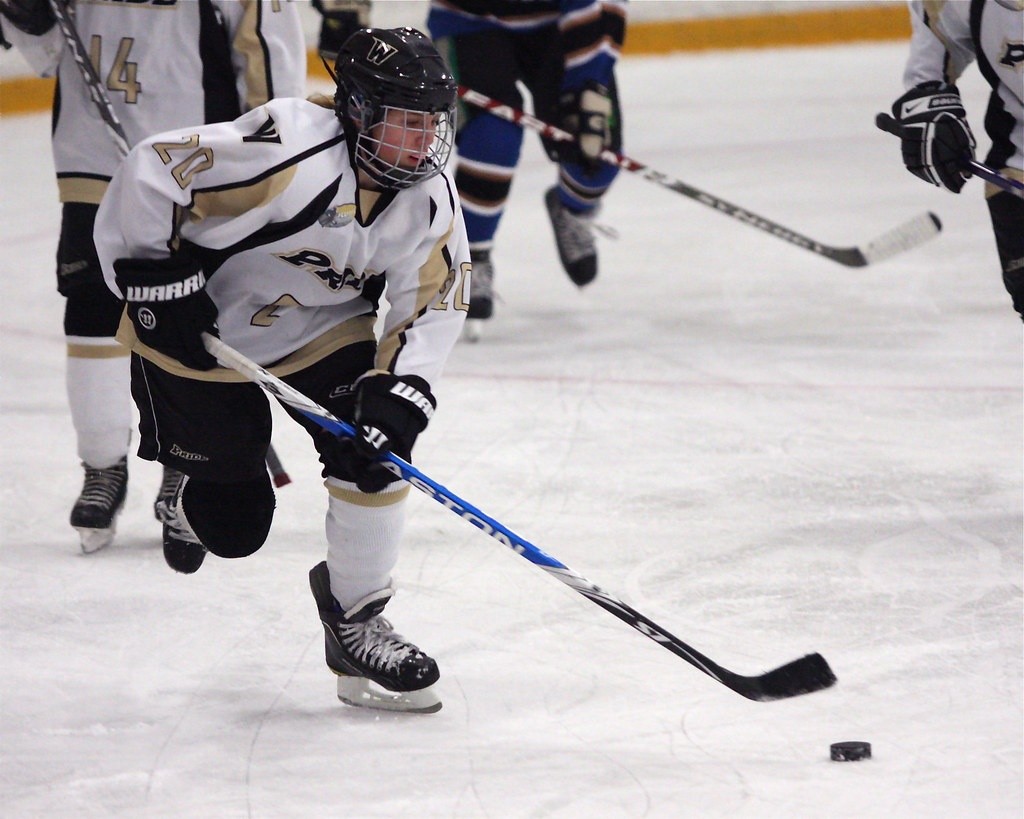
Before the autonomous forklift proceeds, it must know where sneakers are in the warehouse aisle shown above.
[544,186,600,286]
[308,560,443,714]
[465,260,496,319]
[162,476,206,576]
[70,457,129,553]
[152,464,187,519]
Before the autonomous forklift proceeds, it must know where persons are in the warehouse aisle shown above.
[89,25,476,693]
[894,0,1024,328]
[0,0,308,533]
[429,1,630,319]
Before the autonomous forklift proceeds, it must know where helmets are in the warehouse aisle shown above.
[335,27,456,189]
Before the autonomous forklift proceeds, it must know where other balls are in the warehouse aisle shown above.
[830,741,872,762]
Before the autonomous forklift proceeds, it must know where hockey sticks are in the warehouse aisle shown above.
[48,0,134,161]
[452,62,944,271]
[201,328,838,706]
[876,111,1024,202]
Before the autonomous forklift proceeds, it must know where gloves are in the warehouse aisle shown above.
[112,254,221,372]
[892,79,978,194]
[334,373,438,494]
